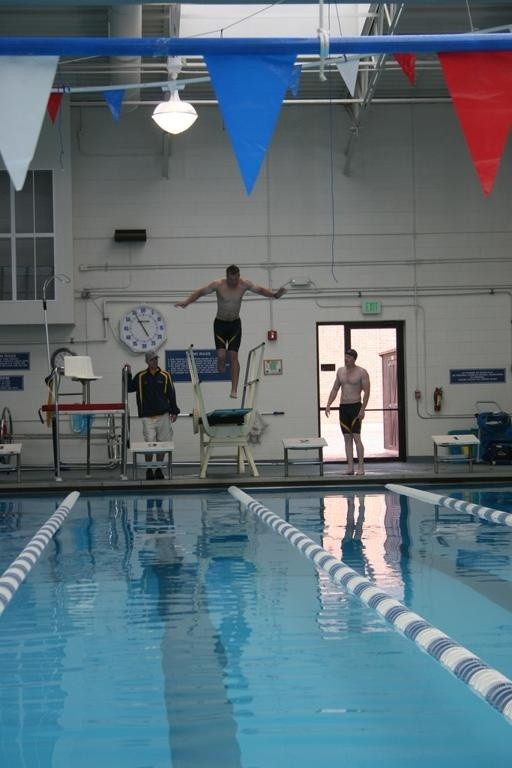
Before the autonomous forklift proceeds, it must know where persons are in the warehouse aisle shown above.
[325,347,371,475]
[123,350,180,480]
[174,264,288,398]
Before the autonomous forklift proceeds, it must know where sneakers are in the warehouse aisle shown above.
[146,468,154,480]
[156,499,162,508]
[146,499,155,508]
[155,468,165,480]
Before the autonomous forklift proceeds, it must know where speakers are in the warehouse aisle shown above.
[115,229,146,242]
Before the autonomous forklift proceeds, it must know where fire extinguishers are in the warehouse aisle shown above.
[434,387,443,411]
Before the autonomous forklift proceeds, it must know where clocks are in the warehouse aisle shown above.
[117,304,171,356]
[49,346,76,373]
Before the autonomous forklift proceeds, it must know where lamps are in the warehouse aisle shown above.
[148,49,200,137]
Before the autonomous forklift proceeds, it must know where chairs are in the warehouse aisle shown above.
[475,411,511,464]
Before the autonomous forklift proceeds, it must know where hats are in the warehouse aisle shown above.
[345,349,358,358]
[145,350,160,361]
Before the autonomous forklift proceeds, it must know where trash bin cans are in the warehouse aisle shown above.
[448,429,478,464]
